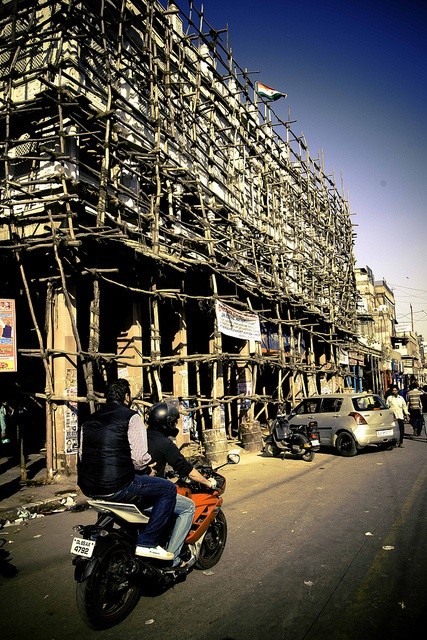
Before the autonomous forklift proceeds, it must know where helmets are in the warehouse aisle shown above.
[147,399,179,438]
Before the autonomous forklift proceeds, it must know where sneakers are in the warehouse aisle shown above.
[136,545,174,560]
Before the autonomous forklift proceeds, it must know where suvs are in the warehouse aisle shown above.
[288,393,399,457]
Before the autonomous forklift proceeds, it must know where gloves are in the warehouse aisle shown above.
[164,465,173,476]
[208,478,218,489]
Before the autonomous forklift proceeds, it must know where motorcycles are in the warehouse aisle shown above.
[263,413,320,462]
[68,454,240,631]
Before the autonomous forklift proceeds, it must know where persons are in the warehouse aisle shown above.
[362,381,427,448]
[146,401,217,570]
[77,379,176,560]
